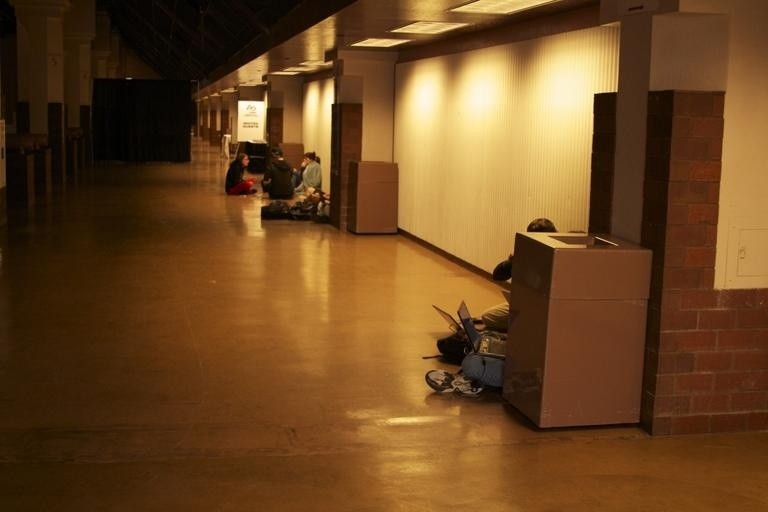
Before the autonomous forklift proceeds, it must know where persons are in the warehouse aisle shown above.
[261,147,322,199]
[226,153,257,195]
[481,218,557,333]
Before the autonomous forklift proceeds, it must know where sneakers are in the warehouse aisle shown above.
[424,369,486,396]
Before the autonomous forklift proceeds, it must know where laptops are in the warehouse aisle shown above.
[458,300,507,360]
[433,305,507,344]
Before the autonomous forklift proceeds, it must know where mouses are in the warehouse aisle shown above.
[472,318,483,324]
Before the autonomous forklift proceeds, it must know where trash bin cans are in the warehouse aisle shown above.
[508,231,655,431]
[348,159,400,236]
[276,141,305,180]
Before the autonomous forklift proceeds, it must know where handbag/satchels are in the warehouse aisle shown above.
[260,188,331,224]
